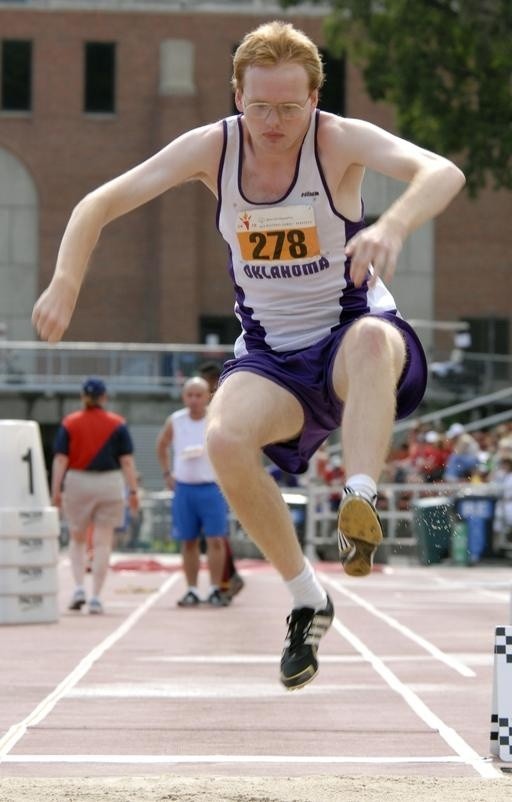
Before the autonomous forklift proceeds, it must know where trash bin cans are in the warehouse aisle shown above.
[454,495,497,566]
[411,495,449,567]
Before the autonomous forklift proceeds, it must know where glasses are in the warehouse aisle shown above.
[242,93,312,122]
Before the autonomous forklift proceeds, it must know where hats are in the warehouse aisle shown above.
[81,379,106,394]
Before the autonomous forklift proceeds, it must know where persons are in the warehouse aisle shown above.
[318,422,512,564]
[32,21,465,692]
[51,378,139,613]
[158,366,241,610]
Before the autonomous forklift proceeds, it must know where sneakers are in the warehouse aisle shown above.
[337,494,383,578]
[69,591,103,614]
[177,573,244,609]
[278,592,335,692]
